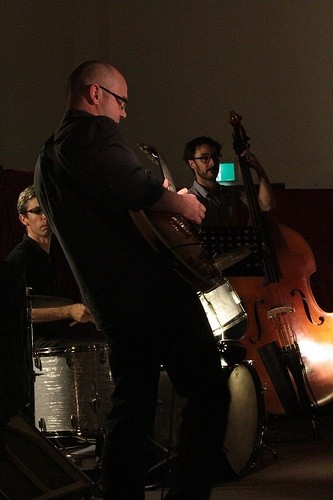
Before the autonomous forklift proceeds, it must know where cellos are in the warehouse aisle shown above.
[218,109,333,434]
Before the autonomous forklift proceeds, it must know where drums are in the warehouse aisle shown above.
[34,341,115,439]
[198,277,248,337]
[151,360,265,481]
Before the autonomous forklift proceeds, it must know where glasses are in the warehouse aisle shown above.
[24,207,44,215]
[194,154,221,163]
[99,86,128,108]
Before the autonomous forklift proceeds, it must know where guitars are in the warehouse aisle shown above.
[129,142,218,291]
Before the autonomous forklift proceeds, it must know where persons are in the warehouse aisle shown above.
[4,185,97,347]
[33,60,232,500]
[182,136,278,227]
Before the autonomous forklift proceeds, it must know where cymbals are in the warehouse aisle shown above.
[25,295,75,309]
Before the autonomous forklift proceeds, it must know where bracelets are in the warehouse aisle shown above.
[258,170,265,177]
